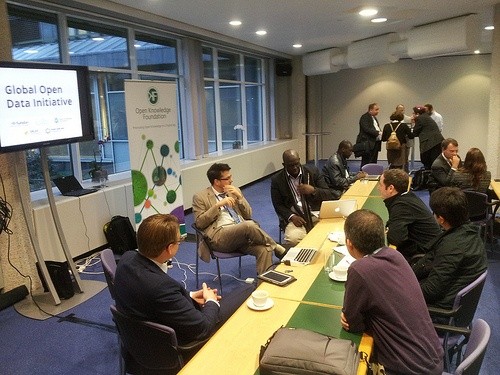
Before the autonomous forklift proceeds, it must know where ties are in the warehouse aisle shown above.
[218,192,240,224]
[448,160,452,166]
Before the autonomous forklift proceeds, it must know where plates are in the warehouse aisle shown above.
[329,272,347,282]
[246,298,274,311]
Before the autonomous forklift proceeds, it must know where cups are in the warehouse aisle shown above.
[360,174,368,183]
[252,290,268,306]
[324,252,335,271]
[333,266,347,278]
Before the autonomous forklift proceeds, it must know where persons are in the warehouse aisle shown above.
[427,138,462,196]
[321,139,368,193]
[192,163,288,289]
[411,186,487,337]
[340,209,445,375]
[377,168,442,264]
[352,103,445,170]
[450,148,491,195]
[113,214,222,362]
[271,150,332,247]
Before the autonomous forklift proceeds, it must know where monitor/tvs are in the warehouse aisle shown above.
[1,61,94,152]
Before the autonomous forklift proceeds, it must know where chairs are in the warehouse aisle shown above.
[110,304,203,375]
[100,249,117,300]
[191,223,257,296]
[361,163,383,175]
[466,191,500,252]
[428,270,491,375]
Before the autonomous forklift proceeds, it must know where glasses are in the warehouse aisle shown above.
[166,234,185,252]
[217,174,232,180]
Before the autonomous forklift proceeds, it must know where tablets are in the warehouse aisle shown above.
[261,271,292,284]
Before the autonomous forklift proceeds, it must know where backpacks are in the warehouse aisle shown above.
[386,122,401,151]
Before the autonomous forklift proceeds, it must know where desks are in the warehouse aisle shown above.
[302,131,332,166]
[177,177,413,375]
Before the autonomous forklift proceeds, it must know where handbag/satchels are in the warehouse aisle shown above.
[103,216,138,255]
[352,141,370,157]
[412,169,433,191]
[258,325,364,375]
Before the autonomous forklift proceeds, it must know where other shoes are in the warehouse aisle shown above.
[274,250,287,260]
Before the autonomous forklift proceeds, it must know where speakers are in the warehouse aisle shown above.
[275,63,291,76]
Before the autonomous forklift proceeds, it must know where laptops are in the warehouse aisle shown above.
[280,233,329,265]
[309,199,357,217]
[54,174,98,198]
[364,163,392,181]
